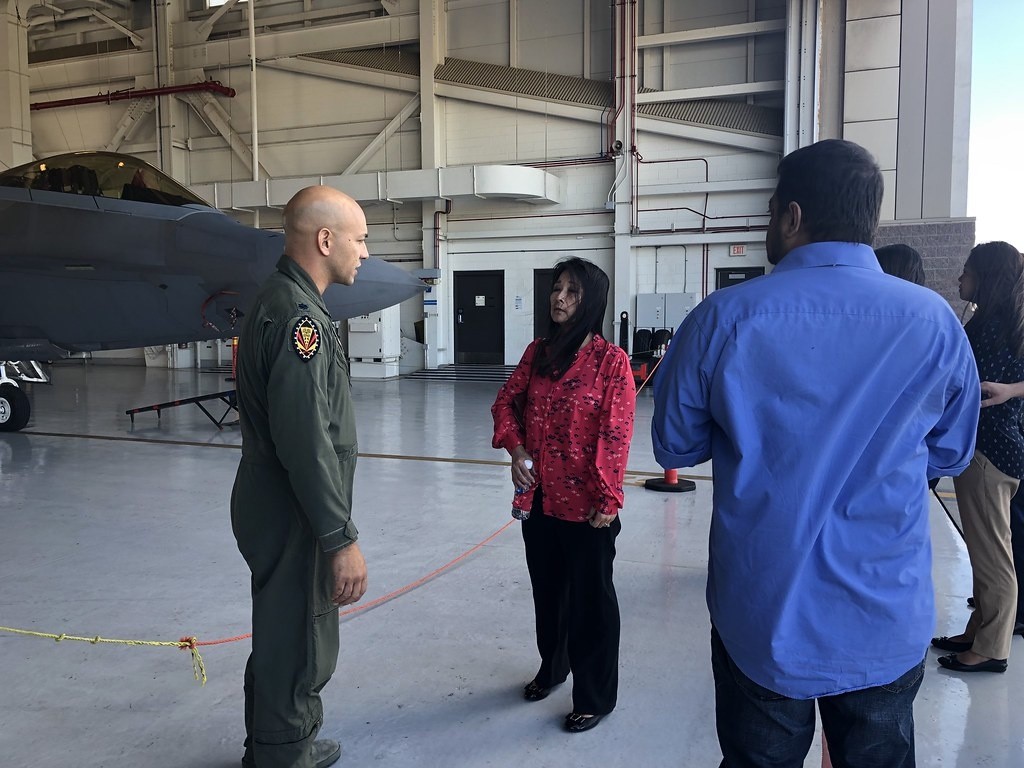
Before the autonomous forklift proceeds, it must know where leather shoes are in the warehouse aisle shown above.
[524,678,553,701]
[938,653,1008,672]
[565,712,605,732]
[931,636,973,653]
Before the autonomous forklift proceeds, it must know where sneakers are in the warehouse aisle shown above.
[311,739,341,768]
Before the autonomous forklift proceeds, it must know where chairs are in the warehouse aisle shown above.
[49,165,100,195]
[630,329,670,385]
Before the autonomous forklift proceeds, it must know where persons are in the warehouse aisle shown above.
[488,257,637,732]
[651,139,982,768]
[229,185,368,768]
[931,241,1024,674]
[874,243,925,287]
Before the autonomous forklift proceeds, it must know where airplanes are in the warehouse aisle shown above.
[0,151,430,433]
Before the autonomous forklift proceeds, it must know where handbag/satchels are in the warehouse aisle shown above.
[512,337,548,436]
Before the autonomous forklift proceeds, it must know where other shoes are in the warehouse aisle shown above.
[968,597,976,607]
[1013,622,1024,635]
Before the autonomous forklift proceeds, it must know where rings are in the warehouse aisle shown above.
[605,522,610,527]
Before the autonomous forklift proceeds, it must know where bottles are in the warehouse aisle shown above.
[511,460,537,521]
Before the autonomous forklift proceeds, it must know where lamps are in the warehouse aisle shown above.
[612,140,624,152]
